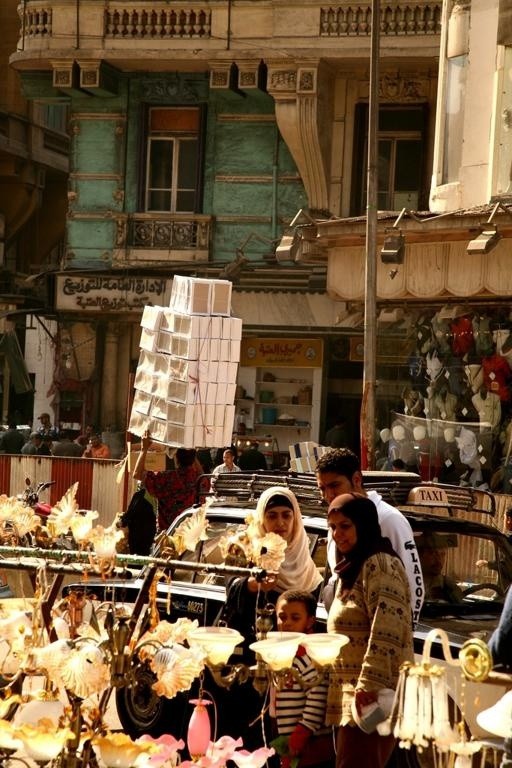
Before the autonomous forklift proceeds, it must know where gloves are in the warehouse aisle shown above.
[287,724,314,758]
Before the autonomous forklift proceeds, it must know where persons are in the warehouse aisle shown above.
[486,585,511,665]
[321,491,416,768]
[241,443,269,469]
[392,458,406,471]
[213,449,242,471]
[1,412,111,459]
[271,588,339,768]
[474,504,511,600]
[316,446,427,629]
[144,447,210,527]
[221,483,324,614]
[236,422,248,434]
[415,530,464,604]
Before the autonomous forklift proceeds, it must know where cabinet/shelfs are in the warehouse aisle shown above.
[253,366,323,429]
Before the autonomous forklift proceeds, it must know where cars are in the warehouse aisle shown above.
[62,509,510,737]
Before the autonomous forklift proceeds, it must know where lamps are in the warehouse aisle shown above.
[0,600,351,768]
[275,202,511,264]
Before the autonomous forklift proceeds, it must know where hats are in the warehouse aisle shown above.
[36,412,50,419]
